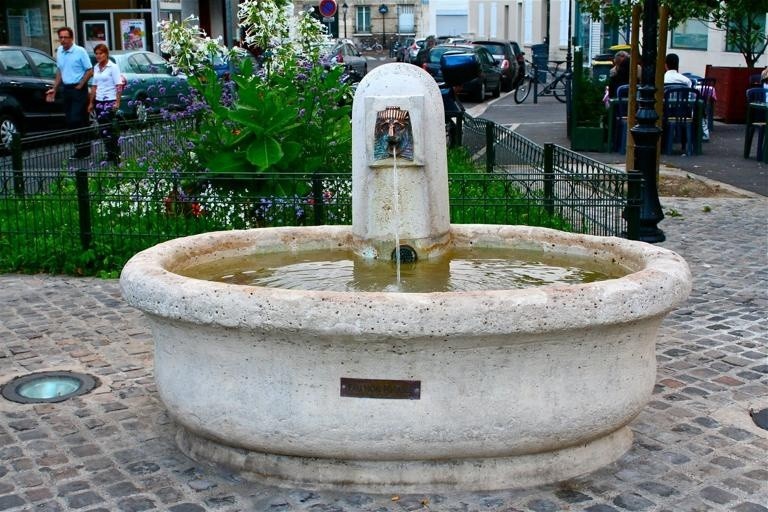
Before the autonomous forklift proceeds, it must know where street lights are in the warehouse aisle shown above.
[378,3,388,50]
[342,3,349,39]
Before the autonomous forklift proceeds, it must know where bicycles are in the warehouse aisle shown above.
[515,52,573,103]
[361,36,382,54]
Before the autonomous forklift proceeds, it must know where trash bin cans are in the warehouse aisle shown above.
[594,54,614,61]
[591,60,613,84]
[609,45,631,56]
[530,43,548,83]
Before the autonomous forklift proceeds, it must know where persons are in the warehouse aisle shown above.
[664,54,693,97]
[88,44,123,163]
[609,55,631,114]
[45,27,93,162]
[761,66,768,103]
[608,52,640,80]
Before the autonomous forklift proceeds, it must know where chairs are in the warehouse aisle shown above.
[605,73,768,162]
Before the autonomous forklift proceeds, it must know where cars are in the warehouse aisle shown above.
[391,32,526,104]
